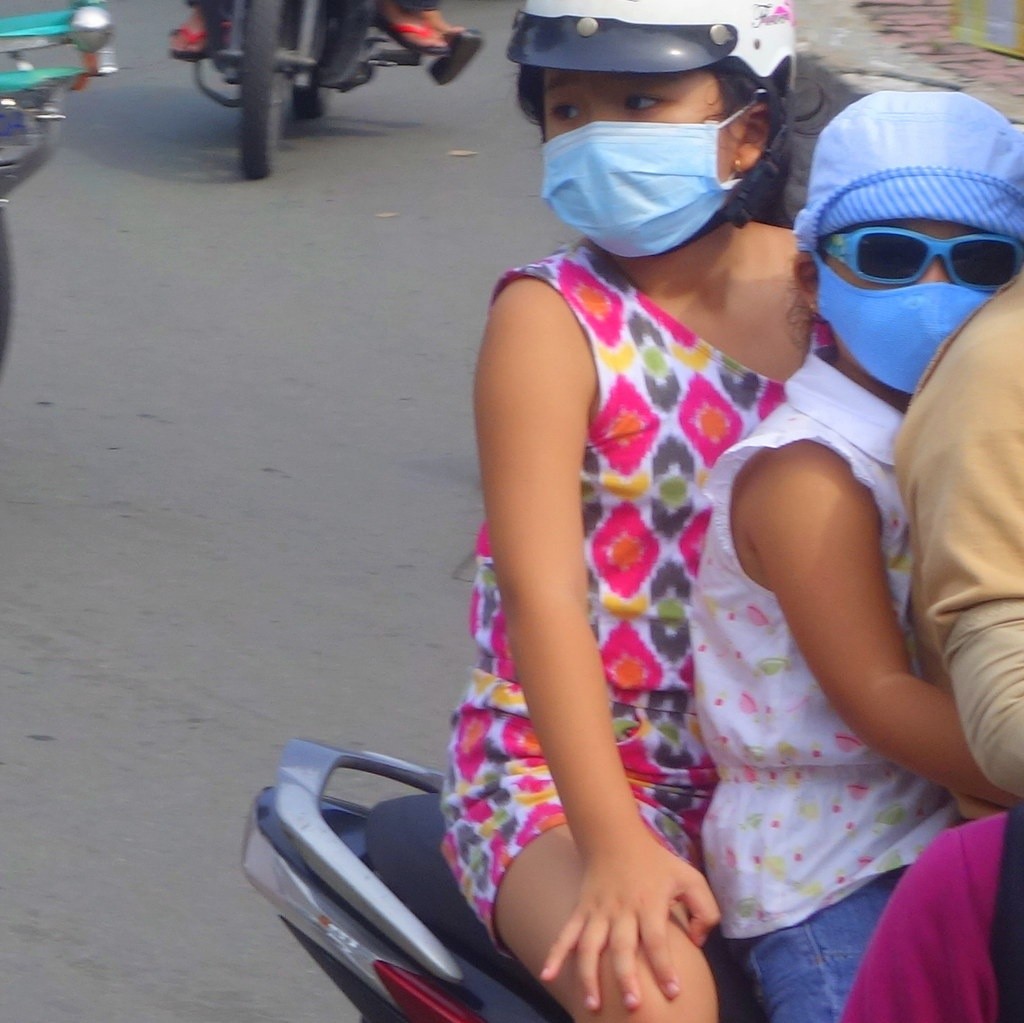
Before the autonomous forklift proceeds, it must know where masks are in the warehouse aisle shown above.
[538,88,763,259]
[808,251,994,395]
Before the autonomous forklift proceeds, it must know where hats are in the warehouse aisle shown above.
[793,89,1023,252]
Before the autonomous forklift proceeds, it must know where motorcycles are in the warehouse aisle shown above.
[183,0,425,181]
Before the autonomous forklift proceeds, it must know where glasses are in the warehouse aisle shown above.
[826,226,1023,292]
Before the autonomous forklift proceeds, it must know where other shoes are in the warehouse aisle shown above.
[430,28,482,85]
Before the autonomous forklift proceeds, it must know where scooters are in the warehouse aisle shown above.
[232,736,779,1023]
[0,0,111,366]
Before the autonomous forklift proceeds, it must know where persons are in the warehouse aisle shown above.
[842,263,1022,1023]
[678,86,1023,1023]
[439,0,832,1023]
[166,0,470,61]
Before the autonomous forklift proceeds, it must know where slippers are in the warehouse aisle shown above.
[169,26,204,59]
[374,16,449,55]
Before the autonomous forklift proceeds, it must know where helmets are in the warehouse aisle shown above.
[505,0,797,98]
